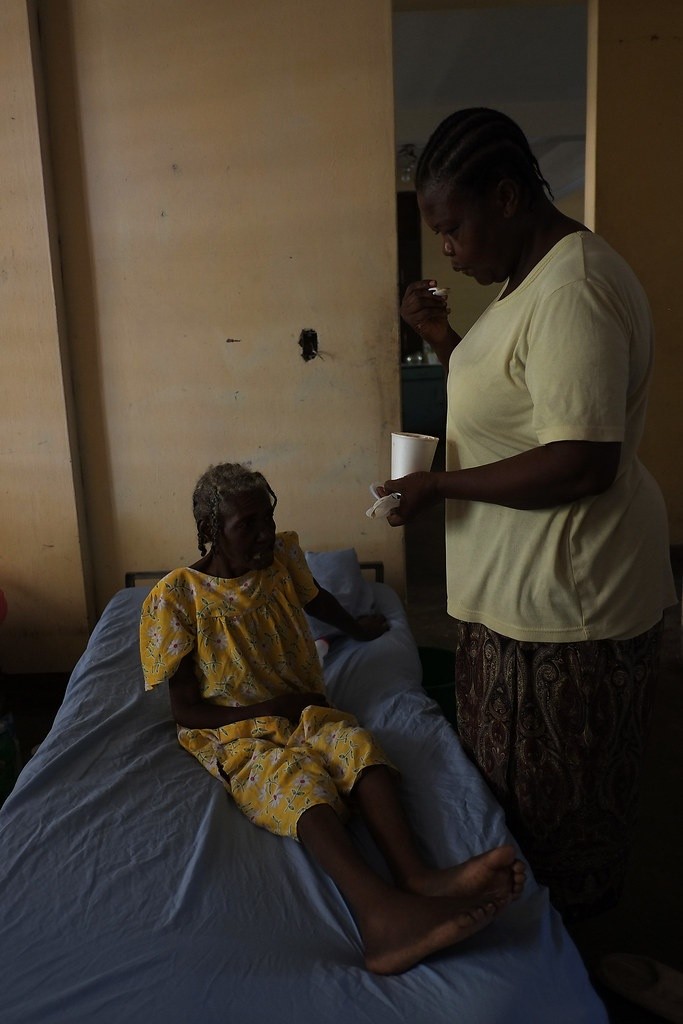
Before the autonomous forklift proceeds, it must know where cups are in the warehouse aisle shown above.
[390,432,439,481]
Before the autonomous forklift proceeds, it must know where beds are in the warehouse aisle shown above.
[0,562,610,1024]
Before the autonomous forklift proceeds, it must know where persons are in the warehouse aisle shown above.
[139,464,527,976]
[376,108,678,924]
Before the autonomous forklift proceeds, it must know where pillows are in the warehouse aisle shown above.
[300,549,373,641]
